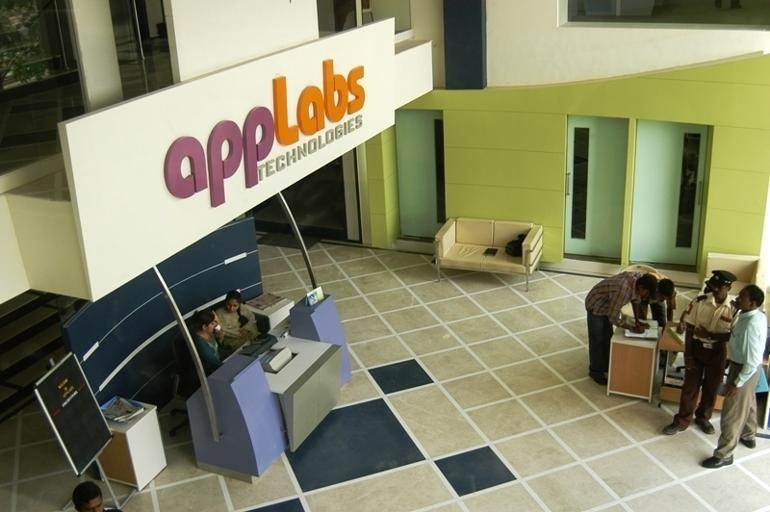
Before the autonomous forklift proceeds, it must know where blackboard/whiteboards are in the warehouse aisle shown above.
[33,349,115,478]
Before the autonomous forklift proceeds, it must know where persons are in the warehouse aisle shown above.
[72,481,123,512]
[584,271,658,385]
[663,270,738,437]
[209,291,262,359]
[174,311,225,398]
[701,284,768,469]
[619,265,677,369]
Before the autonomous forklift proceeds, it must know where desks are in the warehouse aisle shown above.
[94,397,167,492]
[657,320,770,412]
[605,320,663,404]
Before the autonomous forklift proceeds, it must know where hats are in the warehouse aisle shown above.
[712,271,737,284]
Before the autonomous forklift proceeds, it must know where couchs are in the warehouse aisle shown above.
[434,216,544,291]
[700,252,766,312]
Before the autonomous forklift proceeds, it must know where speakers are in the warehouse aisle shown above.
[443,0,490,91]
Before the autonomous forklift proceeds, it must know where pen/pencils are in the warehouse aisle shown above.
[635,316,640,325]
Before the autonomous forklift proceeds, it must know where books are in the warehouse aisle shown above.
[99,394,145,424]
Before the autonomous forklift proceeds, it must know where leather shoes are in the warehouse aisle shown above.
[664,424,687,435]
[695,416,715,434]
[740,438,755,448]
[589,371,607,385]
[703,455,733,468]
